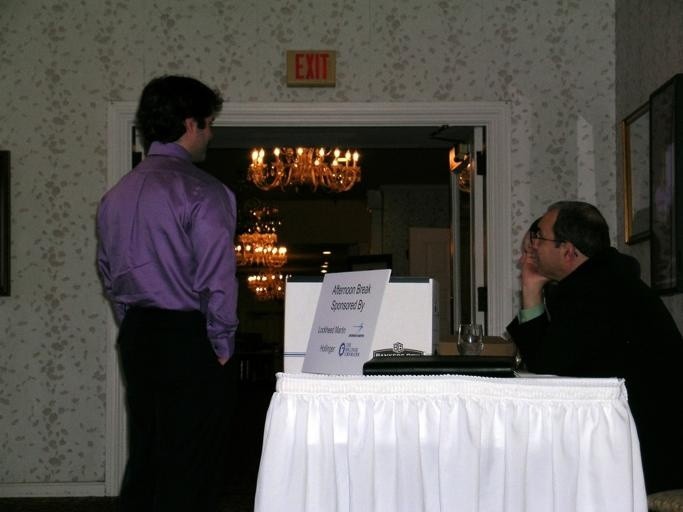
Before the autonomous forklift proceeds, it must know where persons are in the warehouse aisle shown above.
[519,216,550,319]
[95,75,241,511]
[506,200,682,408]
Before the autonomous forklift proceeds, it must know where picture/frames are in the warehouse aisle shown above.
[620,99,653,245]
[648,73,683,296]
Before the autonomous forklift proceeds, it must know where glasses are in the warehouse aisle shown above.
[530,230,567,246]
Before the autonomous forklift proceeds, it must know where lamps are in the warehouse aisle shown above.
[249,145,362,193]
[235,206,287,301]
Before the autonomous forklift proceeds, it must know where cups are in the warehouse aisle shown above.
[457,324,485,355]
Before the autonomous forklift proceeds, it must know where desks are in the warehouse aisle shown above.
[254,374,648,512]
